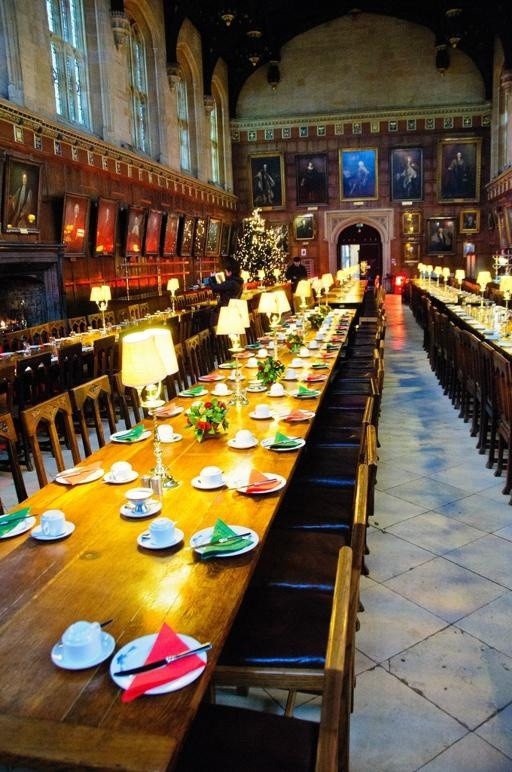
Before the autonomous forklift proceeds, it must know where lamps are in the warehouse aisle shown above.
[216,298,250,406]
[433,266,442,286]
[498,276,512,310]
[89,286,112,334]
[121,327,180,488]
[337,270,346,286]
[417,263,426,280]
[475,271,492,300]
[257,290,291,361]
[166,278,179,314]
[312,278,323,298]
[293,279,312,313]
[258,270,265,289]
[454,269,465,292]
[322,272,334,295]
[240,271,251,293]
[426,265,433,280]
[441,268,450,287]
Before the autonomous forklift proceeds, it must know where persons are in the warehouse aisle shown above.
[12,173,33,227]
[465,212,475,228]
[297,220,312,237]
[285,258,308,291]
[345,154,370,198]
[398,154,421,198]
[210,262,243,315]
[257,162,275,205]
[301,160,321,201]
[431,224,450,248]
[448,151,468,198]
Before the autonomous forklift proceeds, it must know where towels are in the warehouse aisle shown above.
[312,363,329,368]
[307,373,322,381]
[338,326,348,330]
[151,403,176,417]
[121,622,207,703]
[60,461,102,486]
[257,335,273,340]
[329,338,342,342]
[282,323,290,328]
[234,351,249,357]
[183,385,204,397]
[201,373,219,381]
[115,423,144,441]
[342,314,349,318]
[327,344,339,348]
[346,311,354,314]
[270,431,302,449]
[336,330,343,333]
[339,319,349,322]
[323,353,334,358]
[0,507,31,537]
[247,343,259,348]
[284,319,297,323]
[227,361,239,368]
[201,518,254,560]
[246,469,282,493]
[297,386,319,396]
[285,408,312,423]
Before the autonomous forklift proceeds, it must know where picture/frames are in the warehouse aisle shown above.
[294,215,315,241]
[220,223,232,257]
[143,207,167,256]
[387,143,425,203]
[60,190,93,258]
[179,213,198,257]
[1,151,46,234]
[398,210,424,238]
[294,152,329,208]
[435,136,482,204]
[161,211,182,257]
[399,240,422,267]
[338,146,379,202]
[205,215,224,257]
[123,205,149,257]
[246,151,287,211]
[456,207,481,235]
[93,195,121,259]
[425,216,460,256]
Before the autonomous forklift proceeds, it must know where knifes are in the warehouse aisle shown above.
[264,437,302,447]
[114,642,213,677]
[228,478,277,491]
[183,532,251,552]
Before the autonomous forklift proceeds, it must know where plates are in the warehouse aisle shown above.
[288,389,321,399]
[236,472,287,494]
[103,471,139,484]
[287,363,304,368]
[243,344,262,349]
[108,632,208,695]
[148,406,185,417]
[178,390,208,396]
[210,391,233,396]
[189,525,260,558]
[120,499,162,518]
[281,377,300,381]
[289,410,316,421]
[0,512,37,539]
[191,475,227,489]
[311,364,330,368]
[198,375,226,381]
[266,391,287,396]
[31,521,75,540]
[231,353,256,359]
[256,337,276,343]
[159,433,183,442]
[255,354,271,357]
[226,437,260,450]
[296,353,312,357]
[50,632,116,670]
[218,363,242,369]
[108,428,152,443]
[56,466,104,485]
[185,409,191,414]
[260,437,307,452]
[307,346,319,349]
[304,374,328,382]
[245,365,259,368]
[248,411,273,418]
[136,527,184,549]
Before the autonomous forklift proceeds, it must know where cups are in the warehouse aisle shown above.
[199,467,223,485]
[125,488,154,514]
[62,620,102,665]
[269,341,274,347]
[300,349,308,355]
[215,383,227,394]
[192,402,201,406]
[158,424,173,442]
[258,349,267,355]
[287,369,295,378]
[148,518,175,545]
[271,384,283,394]
[292,358,304,366]
[310,340,317,347]
[111,461,132,481]
[41,510,66,536]
[235,429,254,446]
[248,357,257,365]
[255,405,269,417]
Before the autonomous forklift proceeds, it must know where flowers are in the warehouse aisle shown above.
[184,397,232,443]
[256,357,287,386]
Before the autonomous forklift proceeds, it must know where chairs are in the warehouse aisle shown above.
[316,398,374,459]
[364,278,387,316]
[0,365,16,426]
[174,544,355,771]
[459,329,480,437]
[88,311,116,330]
[68,316,87,333]
[115,366,150,429]
[23,391,81,489]
[184,334,210,387]
[339,310,388,377]
[198,328,216,376]
[16,351,56,472]
[211,308,272,367]
[0,308,272,521]
[0,412,29,516]
[69,374,117,458]
[330,377,380,415]
[299,424,378,578]
[492,351,512,506]
[217,463,370,713]
[165,343,190,399]
[58,342,92,450]
[29,324,51,346]
[93,335,119,424]
[478,341,508,469]
[5,328,33,352]
[47,319,68,339]
[420,295,464,419]
